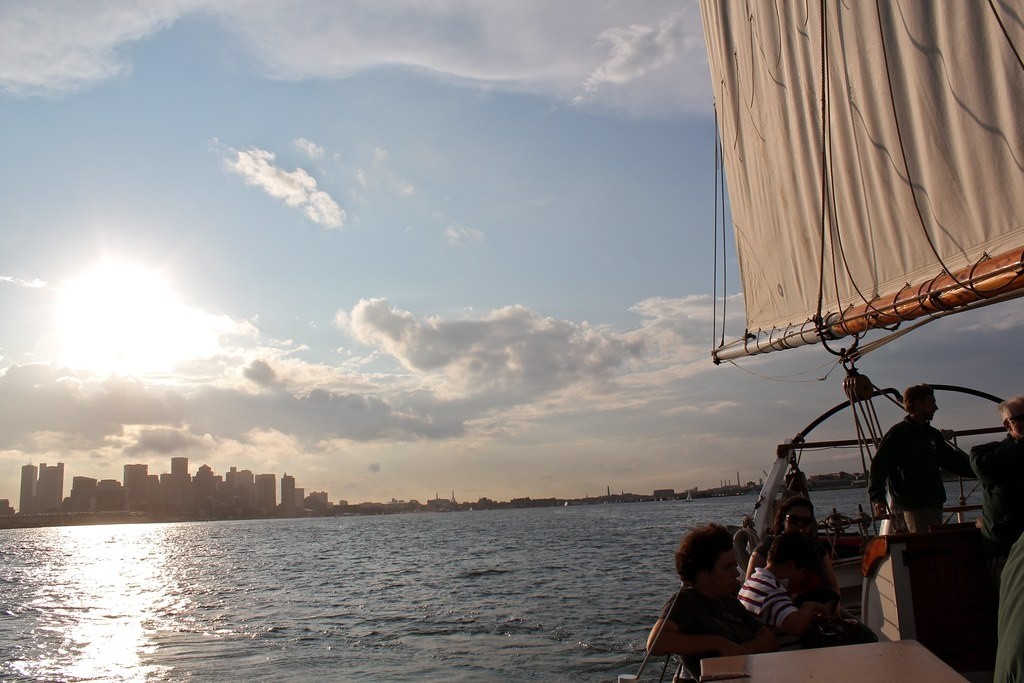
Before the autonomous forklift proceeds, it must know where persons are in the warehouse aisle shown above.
[737,534,828,634]
[744,496,844,626]
[868,383,977,532]
[646,519,796,656]
[969,394,1024,550]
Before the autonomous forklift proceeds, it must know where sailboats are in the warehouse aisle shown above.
[683,490,692,502]
[618,0,1024,683]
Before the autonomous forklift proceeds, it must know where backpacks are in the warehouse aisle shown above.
[802,617,879,650]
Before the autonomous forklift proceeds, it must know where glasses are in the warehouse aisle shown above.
[786,514,812,525]
[1007,413,1023,422]
